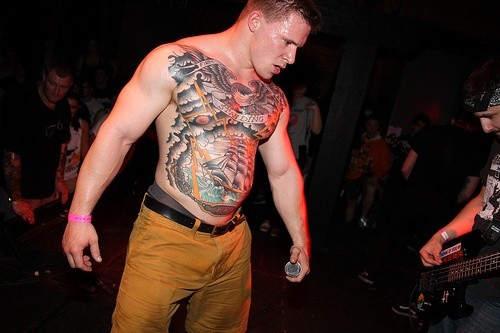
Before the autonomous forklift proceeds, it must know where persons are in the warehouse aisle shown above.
[414,55,500,333]
[60,1,311,333]
[50,60,491,229]
[0,54,70,225]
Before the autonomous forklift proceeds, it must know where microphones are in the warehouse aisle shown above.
[284,261,301,277]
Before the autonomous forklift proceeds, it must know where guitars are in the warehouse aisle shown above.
[409,234,500,326]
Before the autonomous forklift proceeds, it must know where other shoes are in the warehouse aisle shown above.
[270,225,280,237]
[259,218,271,232]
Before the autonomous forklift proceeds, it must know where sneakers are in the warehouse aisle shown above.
[358,271,374,284]
[392,303,417,318]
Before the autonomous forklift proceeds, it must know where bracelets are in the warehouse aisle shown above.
[67,211,93,223]
[436,229,450,241]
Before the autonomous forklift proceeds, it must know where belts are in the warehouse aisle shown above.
[144,195,245,236]
